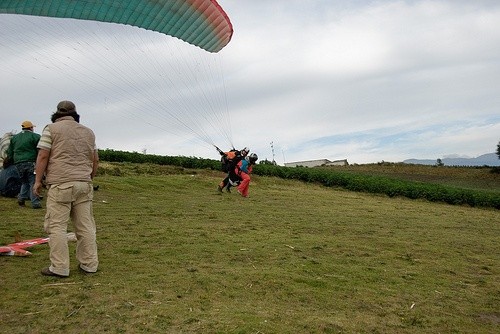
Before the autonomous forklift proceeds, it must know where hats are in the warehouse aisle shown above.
[56,101,75,113]
[21,121,36,128]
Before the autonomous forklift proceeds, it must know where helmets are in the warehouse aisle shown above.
[249,153,258,161]
[243,147,250,155]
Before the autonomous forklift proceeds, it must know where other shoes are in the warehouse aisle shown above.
[33,206,41,208]
[41,268,56,275]
[217,186,223,192]
[19,202,25,206]
[226,188,231,193]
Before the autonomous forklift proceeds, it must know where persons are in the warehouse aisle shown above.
[32,101,100,276]
[217,147,250,193]
[6,121,42,209]
[234,153,258,197]
